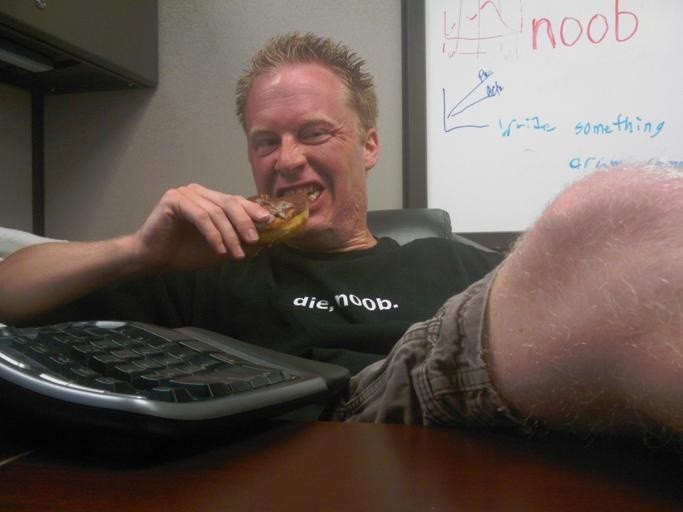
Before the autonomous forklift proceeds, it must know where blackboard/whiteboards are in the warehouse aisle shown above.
[400,1,683,257]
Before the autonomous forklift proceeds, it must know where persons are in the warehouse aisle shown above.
[0,34,682,443]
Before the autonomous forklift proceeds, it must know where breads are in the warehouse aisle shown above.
[245,193,310,259]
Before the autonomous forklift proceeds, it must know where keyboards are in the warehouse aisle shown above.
[1,319,351,460]
